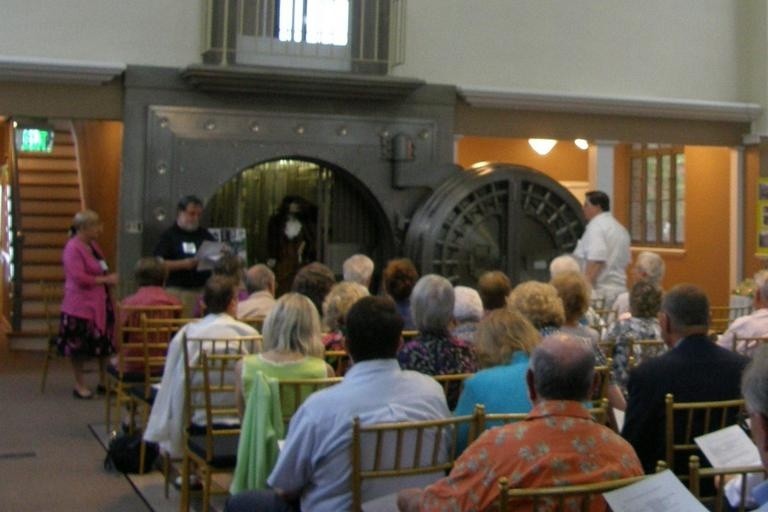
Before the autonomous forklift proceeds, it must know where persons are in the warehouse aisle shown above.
[154,194,216,289]
[264,195,310,268]
[60,209,120,400]
[111,251,768,510]
[572,190,633,299]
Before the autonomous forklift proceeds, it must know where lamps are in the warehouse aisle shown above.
[527,137,556,155]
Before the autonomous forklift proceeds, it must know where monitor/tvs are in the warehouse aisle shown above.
[13,126,55,154]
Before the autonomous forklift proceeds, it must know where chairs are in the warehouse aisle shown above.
[34,274,768,512]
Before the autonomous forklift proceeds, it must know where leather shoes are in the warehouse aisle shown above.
[73,386,94,400]
[96,384,118,398]
